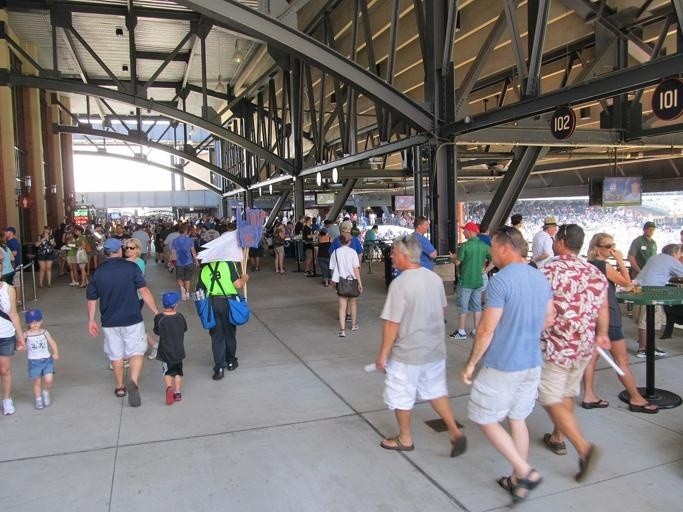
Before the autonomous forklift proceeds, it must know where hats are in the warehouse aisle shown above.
[3,226,16,233]
[102,237,125,253]
[643,221,658,229]
[24,307,44,324]
[539,215,560,228]
[459,222,480,233]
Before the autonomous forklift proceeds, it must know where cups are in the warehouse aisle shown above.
[631,280,642,294]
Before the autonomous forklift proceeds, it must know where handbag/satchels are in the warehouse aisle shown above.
[194,297,218,330]
[226,294,251,326]
[336,274,361,298]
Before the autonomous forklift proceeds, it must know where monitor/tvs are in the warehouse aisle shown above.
[316,192,335,205]
[395,195,415,211]
[600,175,641,207]
[587,175,602,206]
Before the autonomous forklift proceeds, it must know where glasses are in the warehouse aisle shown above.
[125,246,140,250]
[499,225,517,248]
[597,243,617,249]
[401,235,409,250]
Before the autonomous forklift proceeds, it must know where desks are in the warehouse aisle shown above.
[611,281,682,411]
[290,238,322,279]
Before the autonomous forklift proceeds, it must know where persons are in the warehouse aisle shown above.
[603,182,623,201]
[624,179,641,200]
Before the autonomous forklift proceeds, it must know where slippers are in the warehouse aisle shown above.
[580,397,610,409]
[379,434,416,452]
[448,434,468,460]
[626,400,660,414]
[320,280,333,288]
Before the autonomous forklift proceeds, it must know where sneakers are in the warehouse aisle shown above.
[155,258,165,264]
[164,386,175,405]
[108,357,130,370]
[2,397,16,416]
[147,341,161,361]
[226,358,239,371]
[467,329,476,338]
[570,444,602,483]
[210,367,225,381]
[350,325,360,332]
[180,286,187,302]
[34,397,43,410]
[42,390,51,407]
[250,264,314,278]
[655,347,667,356]
[337,330,346,337]
[635,349,659,359]
[540,431,567,455]
[337,314,354,322]
[172,393,182,401]
[38,270,90,290]
[185,291,191,300]
[448,329,467,340]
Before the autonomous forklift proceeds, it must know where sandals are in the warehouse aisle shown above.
[112,386,126,398]
[494,475,514,492]
[506,468,545,509]
[126,381,142,407]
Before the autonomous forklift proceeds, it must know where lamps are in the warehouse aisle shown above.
[12,175,84,205]
[213,51,226,93]
[227,29,245,67]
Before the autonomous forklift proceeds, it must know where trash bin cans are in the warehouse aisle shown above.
[383,246,402,286]
